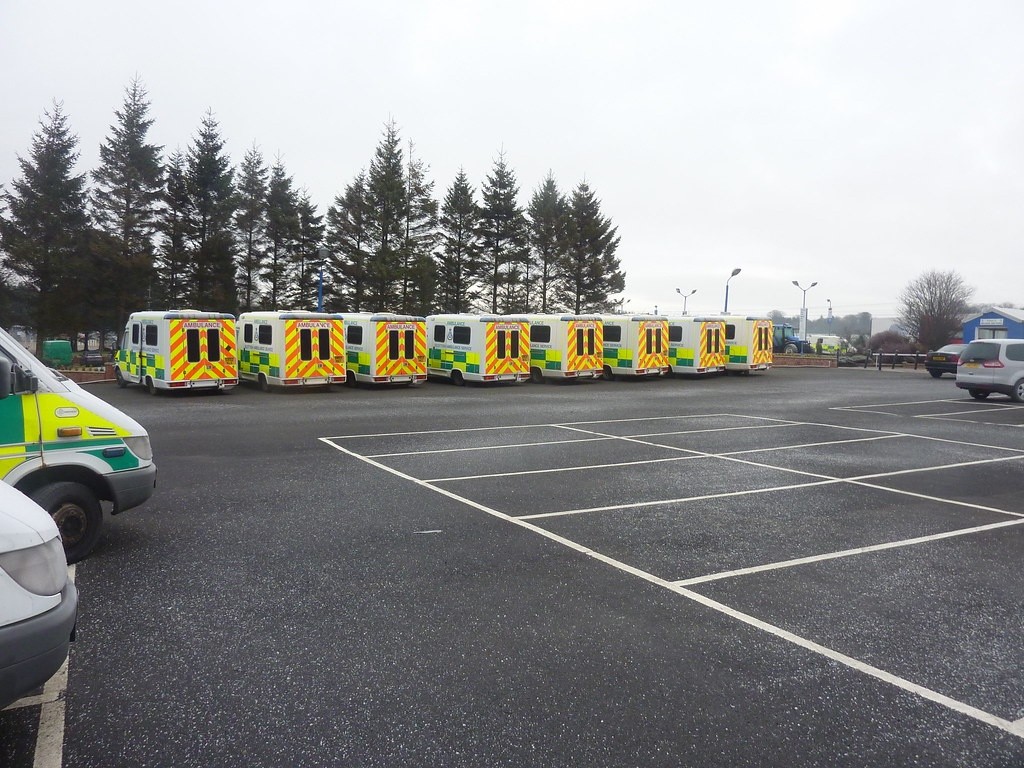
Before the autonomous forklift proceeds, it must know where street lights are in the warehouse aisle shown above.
[792,280,818,354]
[724,268,742,312]
[676,288,697,316]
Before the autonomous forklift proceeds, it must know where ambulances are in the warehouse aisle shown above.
[598,315,669,381]
[337,313,428,387]
[722,316,773,375]
[668,317,725,378]
[114,310,239,395]
[1,326,158,567]
[426,314,530,386]
[527,314,604,385]
[236,310,347,393]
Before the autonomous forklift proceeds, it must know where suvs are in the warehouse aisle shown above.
[772,324,811,354]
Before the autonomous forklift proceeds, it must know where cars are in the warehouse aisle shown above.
[0,474,80,708]
[924,344,970,377]
[956,338,1024,402]
